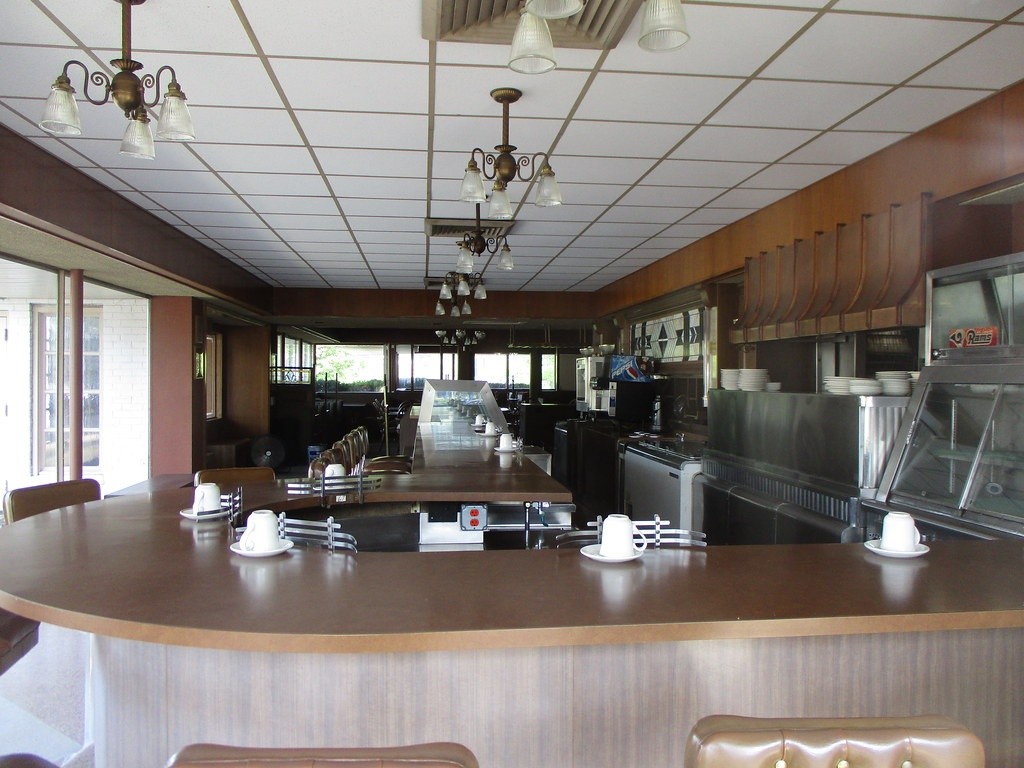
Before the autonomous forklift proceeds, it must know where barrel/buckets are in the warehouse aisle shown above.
[309,446,324,463]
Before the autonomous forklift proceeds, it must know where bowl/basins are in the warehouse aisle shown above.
[600,344,616,354]
[581,348,594,357]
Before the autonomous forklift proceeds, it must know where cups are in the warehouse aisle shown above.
[485,422,494,434]
[460,401,473,416]
[240,510,278,551]
[599,514,647,557]
[475,415,485,424]
[879,511,920,552]
[325,464,347,487]
[500,434,511,449]
[192,483,220,515]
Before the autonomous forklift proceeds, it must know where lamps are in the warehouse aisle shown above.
[459,89,562,219]
[456,196,513,274]
[508,0,687,73]
[434,287,471,316]
[39,1,195,159]
[440,271,487,299]
[432,323,485,351]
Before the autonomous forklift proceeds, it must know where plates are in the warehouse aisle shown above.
[480,434,498,436]
[580,544,643,563]
[230,539,294,557]
[313,485,354,490]
[766,382,781,391]
[823,376,883,395]
[180,508,228,519]
[864,539,930,558]
[494,447,518,452]
[875,371,920,396]
[474,430,485,433]
[470,423,486,426]
[720,368,768,390]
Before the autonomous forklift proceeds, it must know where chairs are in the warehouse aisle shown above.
[557,512,707,551]
[227,487,244,523]
[234,512,357,554]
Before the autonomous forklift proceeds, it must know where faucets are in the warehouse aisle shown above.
[676,432,685,442]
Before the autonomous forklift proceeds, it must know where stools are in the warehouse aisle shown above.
[174,741,479,768]
[308,426,411,481]
[193,467,276,490]
[6,479,101,524]
[682,715,988,768]
[0,607,40,675]
